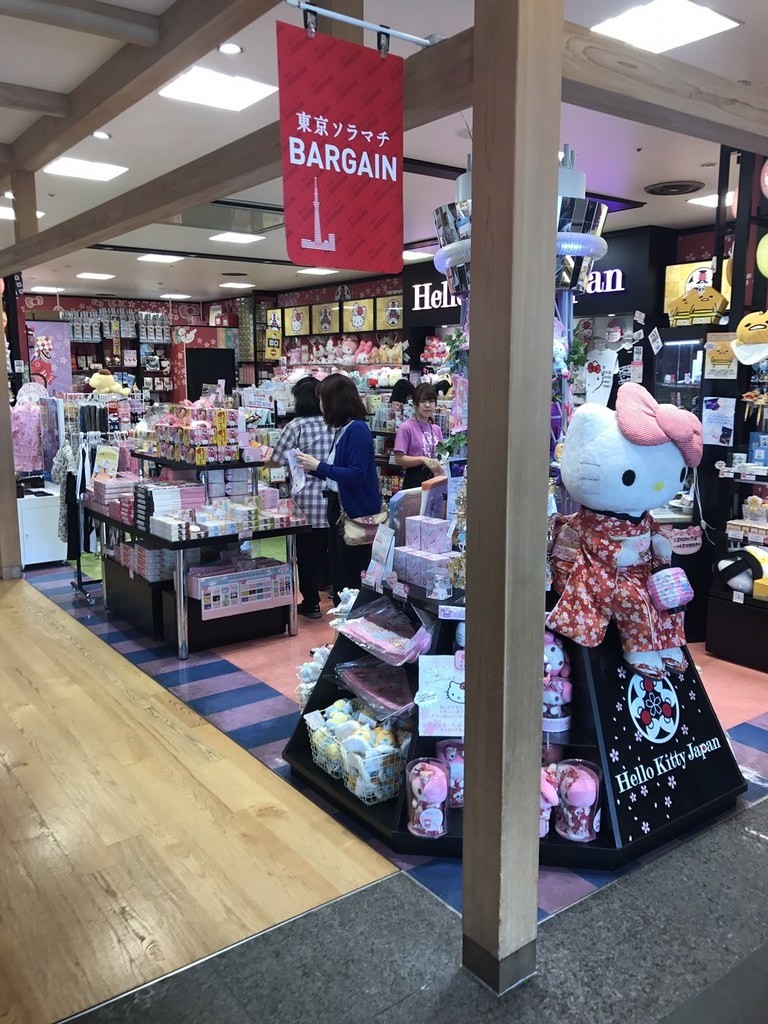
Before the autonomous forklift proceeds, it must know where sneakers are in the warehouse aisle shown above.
[297,602,322,619]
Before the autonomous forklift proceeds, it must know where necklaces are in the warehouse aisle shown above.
[415,415,435,459]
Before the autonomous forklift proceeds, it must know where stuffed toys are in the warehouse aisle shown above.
[540,743,596,841]
[296,645,331,710]
[275,335,453,391]
[545,382,703,681]
[310,700,408,800]
[408,762,446,836]
[89,370,132,397]
[436,749,465,807]
[542,632,572,720]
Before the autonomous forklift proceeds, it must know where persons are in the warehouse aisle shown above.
[387,379,416,404]
[262,378,343,617]
[393,384,449,491]
[442,211,456,244]
[294,373,382,607]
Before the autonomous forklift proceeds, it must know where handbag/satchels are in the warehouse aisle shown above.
[339,510,390,546]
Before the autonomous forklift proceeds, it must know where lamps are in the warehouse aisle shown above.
[53,291,64,313]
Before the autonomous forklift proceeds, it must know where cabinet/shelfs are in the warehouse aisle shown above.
[238,330,402,428]
[357,388,467,517]
[281,487,749,869]
[16,487,68,570]
[84,450,312,660]
[654,324,768,673]
[71,321,175,408]
